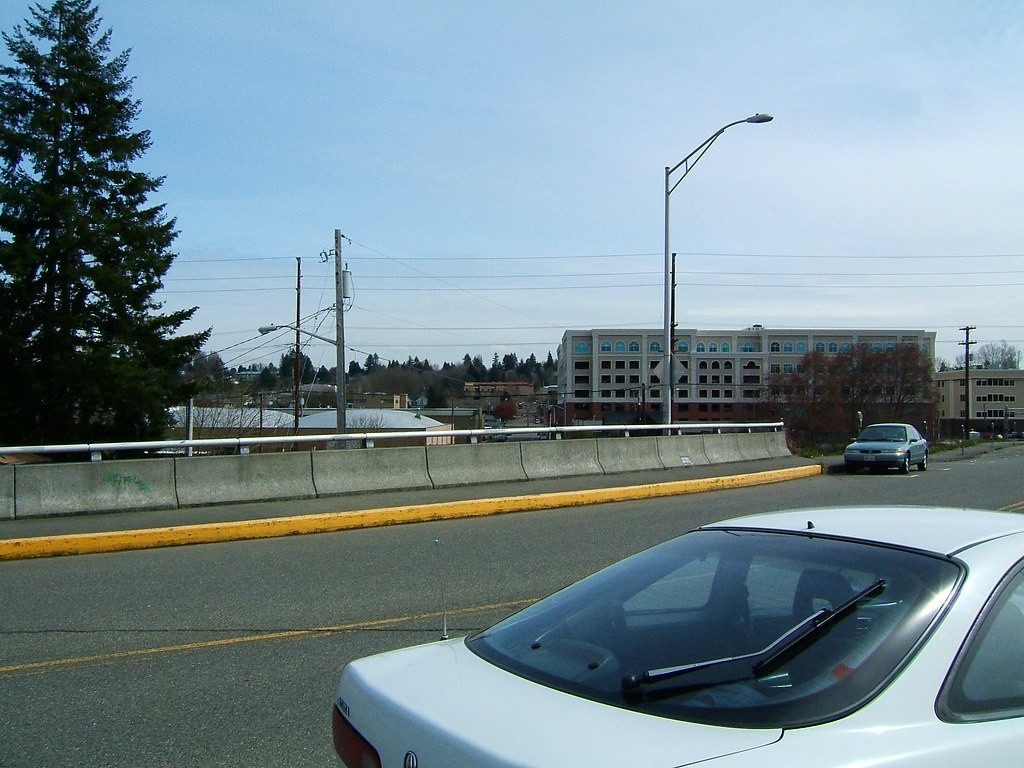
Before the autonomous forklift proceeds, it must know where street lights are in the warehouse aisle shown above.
[258,324,346,436]
[663,113,775,431]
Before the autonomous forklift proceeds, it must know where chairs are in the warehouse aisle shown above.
[793,568,859,670]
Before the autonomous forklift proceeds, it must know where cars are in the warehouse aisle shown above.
[844,423,930,474]
[517,400,541,424]
[980,426,1022,442]
[332,505,1024,768]
[242,390,305,409]
[482,421,507,441]
